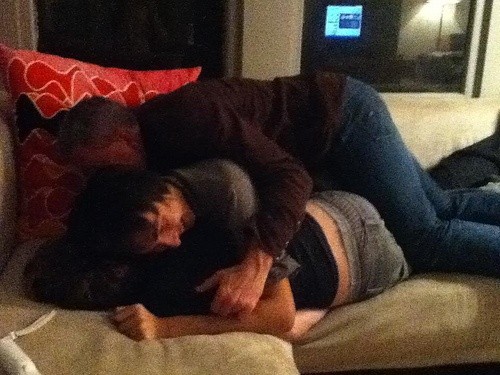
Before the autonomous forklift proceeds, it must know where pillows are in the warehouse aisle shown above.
[0,42,203,244]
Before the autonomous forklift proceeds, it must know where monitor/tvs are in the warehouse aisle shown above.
[324,5,363,38]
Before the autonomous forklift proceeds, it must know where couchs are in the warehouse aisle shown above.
[0,93,500,375]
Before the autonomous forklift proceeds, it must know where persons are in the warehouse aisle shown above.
[20,69,500,340]
